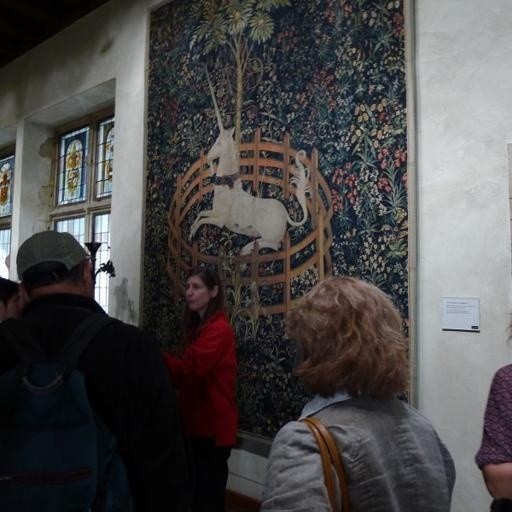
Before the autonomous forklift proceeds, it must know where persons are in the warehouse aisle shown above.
[0,230,177,512]
[160,265,242,512]
[473,307,512,512]
[0,275,27,323]
[258,271,458,512]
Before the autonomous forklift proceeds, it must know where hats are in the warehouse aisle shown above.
[16,230,94,277]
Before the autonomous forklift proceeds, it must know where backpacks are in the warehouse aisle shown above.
[0,314,137,512]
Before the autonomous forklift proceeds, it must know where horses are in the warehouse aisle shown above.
[188,126,312,258]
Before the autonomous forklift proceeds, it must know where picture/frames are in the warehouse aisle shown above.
[137,1,420,459]
